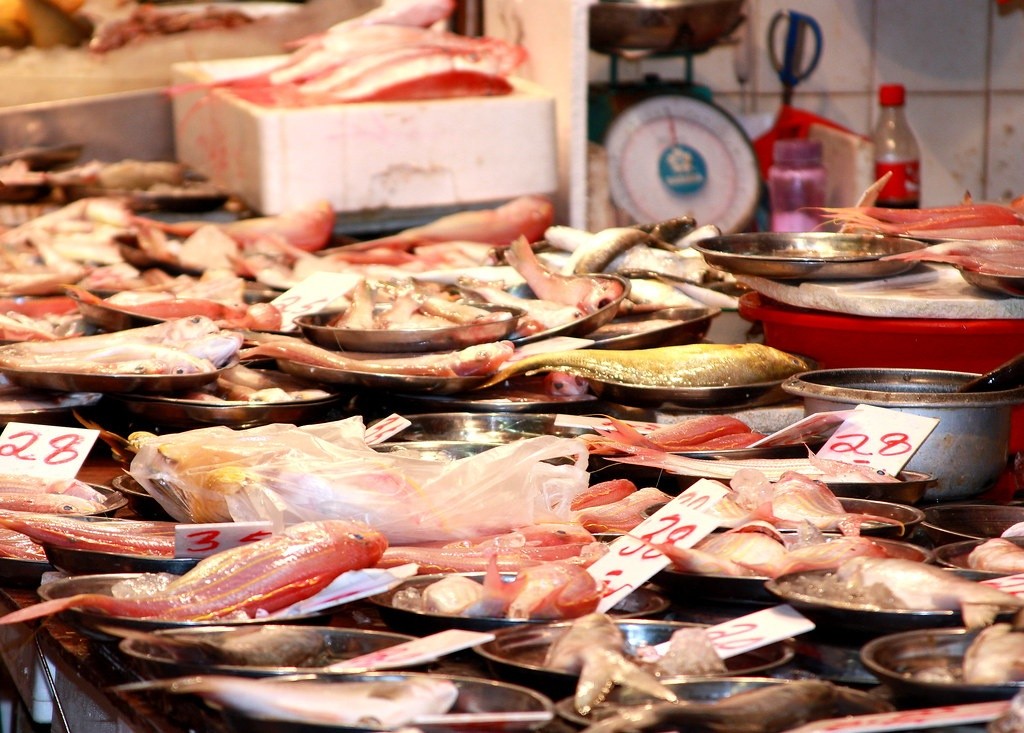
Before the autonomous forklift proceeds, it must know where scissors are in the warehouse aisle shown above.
[767,9,824,106]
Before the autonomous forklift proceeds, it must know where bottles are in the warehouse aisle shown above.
[763,139,826,234]
[871,82,918,210]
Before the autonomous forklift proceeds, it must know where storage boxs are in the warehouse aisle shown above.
[172,61,558,216]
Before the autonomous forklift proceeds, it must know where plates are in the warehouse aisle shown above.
[588,0,747,54]
[0,152,1024,733]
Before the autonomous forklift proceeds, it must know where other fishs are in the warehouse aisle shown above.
[2,1,1023,731]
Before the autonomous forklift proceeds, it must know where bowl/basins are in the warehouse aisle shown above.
[730,283,1024,456]
[780,361,1020,499]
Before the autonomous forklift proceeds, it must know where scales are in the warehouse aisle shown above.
[587,0,762,235]
